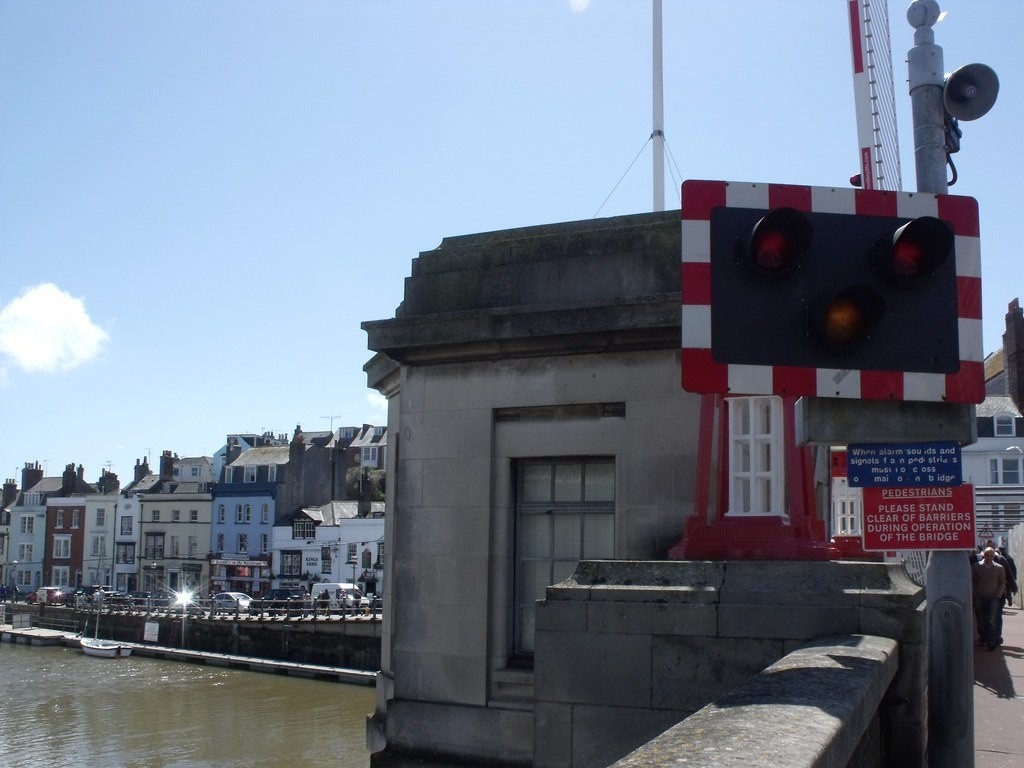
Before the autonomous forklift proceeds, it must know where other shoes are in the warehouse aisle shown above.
[980,641,987,647]
[1008,600,1012,606]
[987,643,996,650]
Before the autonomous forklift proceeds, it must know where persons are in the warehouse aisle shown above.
[339,590,347,615]
[208,591,216,607]
[351,589,365,617]
[93,587,105,611]
[969,540,1018,651]
[13,585,19,602]
[0,585,7,602]
[303,589,330,618]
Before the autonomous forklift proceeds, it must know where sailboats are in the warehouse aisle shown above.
[80,537,132,658]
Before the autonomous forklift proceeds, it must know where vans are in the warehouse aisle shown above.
[310,583,369,615]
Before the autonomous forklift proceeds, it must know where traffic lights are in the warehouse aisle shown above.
[710,205,963,375]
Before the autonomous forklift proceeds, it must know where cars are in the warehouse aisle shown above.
[209,592,253,615]
[65,586,198,613]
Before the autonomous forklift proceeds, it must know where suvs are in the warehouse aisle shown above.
[249,588,311,617]
[25,587,66,606]
